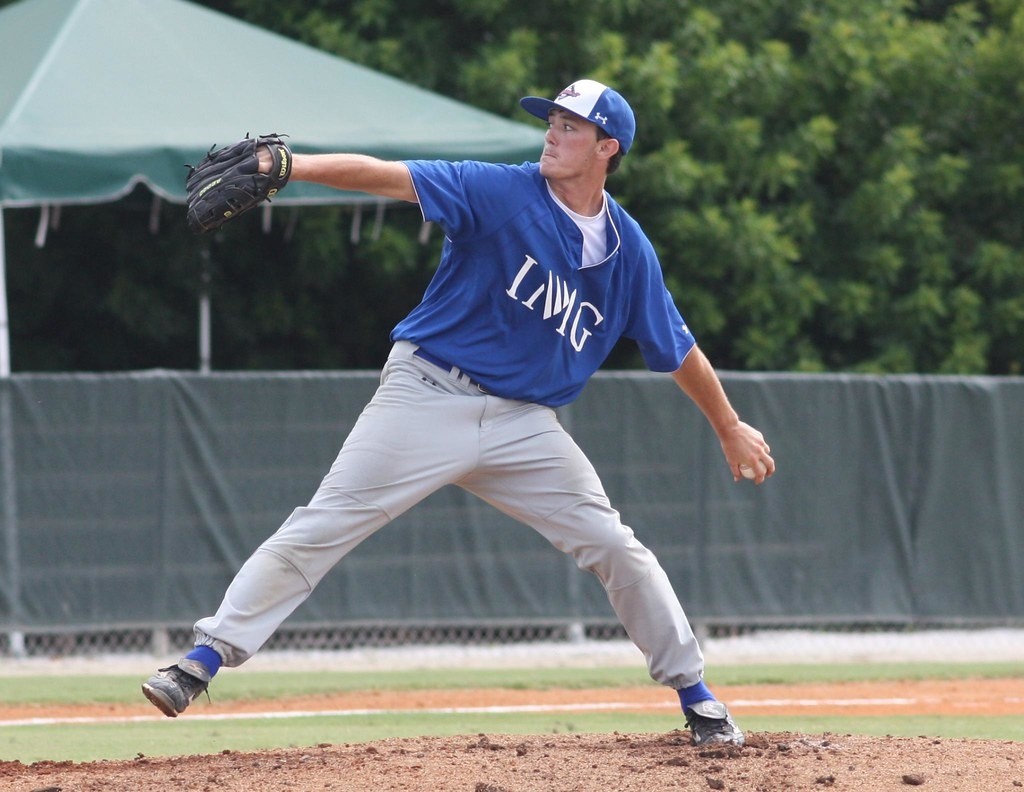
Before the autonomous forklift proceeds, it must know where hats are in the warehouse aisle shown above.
[520,78,635,155]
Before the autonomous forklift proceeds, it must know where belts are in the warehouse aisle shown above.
[414,347,496,397]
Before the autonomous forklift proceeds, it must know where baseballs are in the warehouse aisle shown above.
[738,459,767,480]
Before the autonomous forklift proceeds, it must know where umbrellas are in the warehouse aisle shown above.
[0,0,552,375]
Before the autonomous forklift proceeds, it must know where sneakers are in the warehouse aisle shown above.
[685,701,745,748]
[142,657,213,718]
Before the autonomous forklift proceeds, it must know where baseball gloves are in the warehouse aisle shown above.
[182,131,291,232]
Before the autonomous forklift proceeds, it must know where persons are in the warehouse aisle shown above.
[142,78,774,745]
